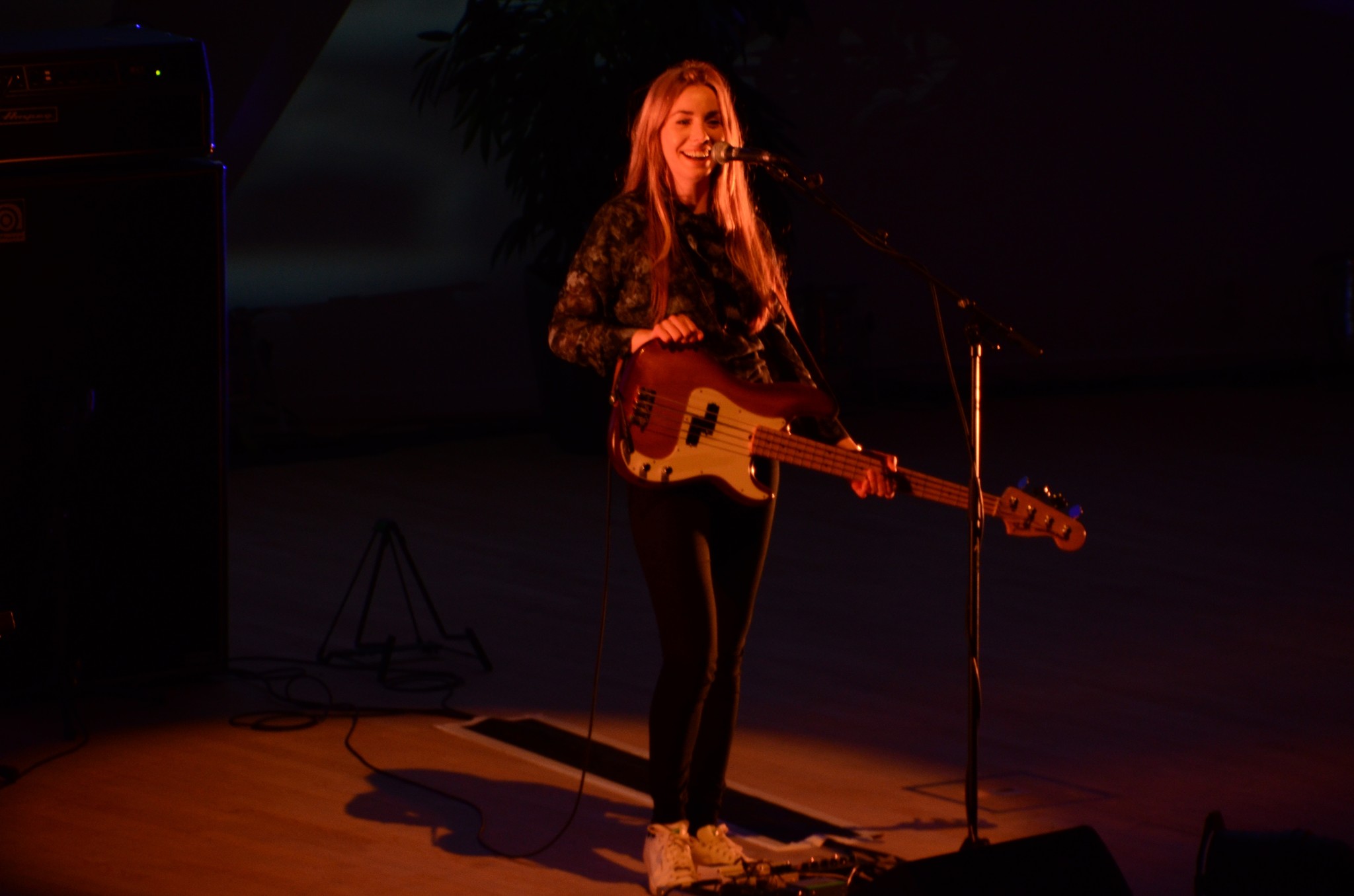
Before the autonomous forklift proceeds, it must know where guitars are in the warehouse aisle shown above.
[608,332,1087,553]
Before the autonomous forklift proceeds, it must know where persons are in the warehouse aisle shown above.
[545,59,901,894]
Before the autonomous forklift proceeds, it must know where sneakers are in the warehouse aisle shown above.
[688,823,770,876]
[644,821,698,895]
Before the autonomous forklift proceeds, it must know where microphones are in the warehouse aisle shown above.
[714,141,780,164]
